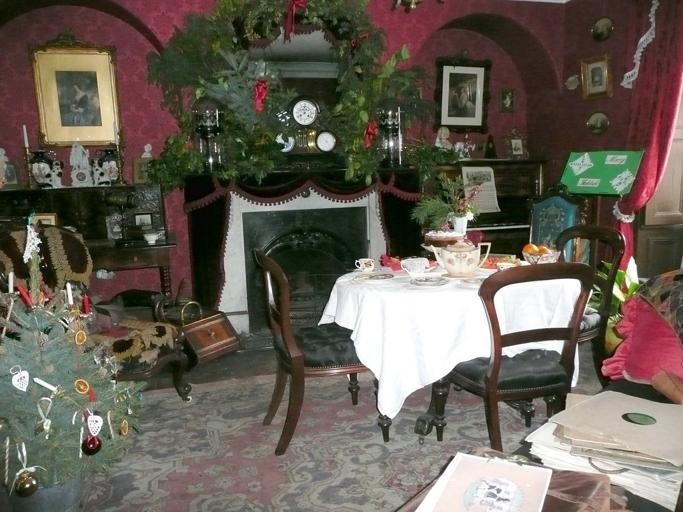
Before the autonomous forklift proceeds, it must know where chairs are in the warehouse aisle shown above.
[254,248,392,455]
[555,223,626,391]
[0,223,197,434]
[432,262,594,454]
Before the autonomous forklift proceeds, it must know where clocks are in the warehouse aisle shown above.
[272,96,337,154]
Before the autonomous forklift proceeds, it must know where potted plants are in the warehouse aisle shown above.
[410,178,485,234]
[0,321,149,512]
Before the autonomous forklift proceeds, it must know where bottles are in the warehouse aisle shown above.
[95,148,119,185]
[29,150,52,187]
[484,134,496,159]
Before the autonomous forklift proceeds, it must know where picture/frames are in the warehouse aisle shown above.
[433,55,492,134]
[105,215,123,240]
[498,86,516,113]
[504,131,530,160]
[585,111,610,134]
[132,157,154,185]
[28,38,125,149]
[133,213,153,226]
[29,212,58,226]
[580,53,614,100]
[0,162,25,190]
[591,18,614,40]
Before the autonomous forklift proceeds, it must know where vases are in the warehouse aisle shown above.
[603,322,625,361]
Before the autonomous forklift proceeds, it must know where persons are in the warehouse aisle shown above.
[455,87,471,116]
[62,80,89,126]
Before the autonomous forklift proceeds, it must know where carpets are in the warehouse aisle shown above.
[0,338,683,512]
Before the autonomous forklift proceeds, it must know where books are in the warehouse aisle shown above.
[411,451,555,512]
[524,391,682,511]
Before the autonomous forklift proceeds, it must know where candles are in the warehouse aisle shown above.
[22,125,29,148]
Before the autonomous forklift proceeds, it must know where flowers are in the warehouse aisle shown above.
[595,254,641,325]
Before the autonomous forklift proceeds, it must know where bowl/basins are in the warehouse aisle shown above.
[478,253,517,271]
[522,250,561,264]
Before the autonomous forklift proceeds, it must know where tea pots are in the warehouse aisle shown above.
[431,237,494,278]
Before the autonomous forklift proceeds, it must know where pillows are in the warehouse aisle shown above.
[601,292,682,385]
[635,268,683,344]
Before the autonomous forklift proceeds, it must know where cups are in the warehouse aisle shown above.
[496,262,517,273]
[400,258,425,278]
[143,234,162,245]
[353,258,375,274]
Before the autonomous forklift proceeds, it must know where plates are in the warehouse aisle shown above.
[114,238,146,248]
[411,278,449,286]
[369,274,393,279]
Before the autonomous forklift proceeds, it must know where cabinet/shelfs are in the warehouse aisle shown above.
[0,181,175,308]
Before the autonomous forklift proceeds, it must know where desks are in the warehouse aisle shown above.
[409,157,549,232]
